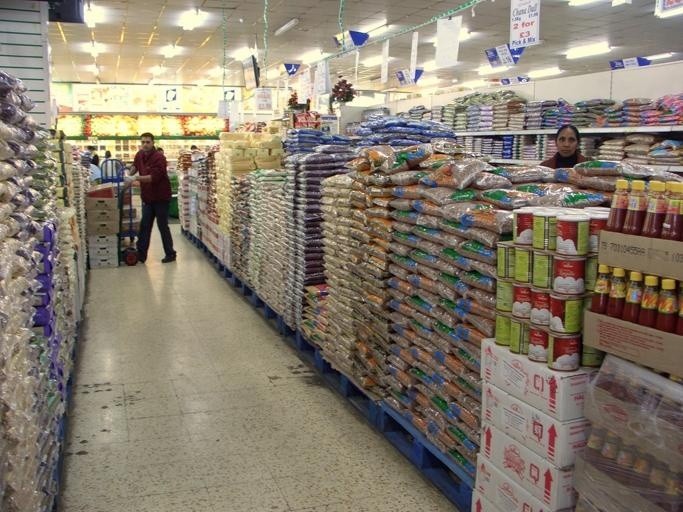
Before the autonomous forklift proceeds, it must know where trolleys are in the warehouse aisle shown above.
[100,159,138,266]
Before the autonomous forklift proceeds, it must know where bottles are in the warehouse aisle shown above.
[593,177,683,338]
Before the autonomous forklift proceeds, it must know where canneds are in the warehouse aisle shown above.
[497,207,610,371]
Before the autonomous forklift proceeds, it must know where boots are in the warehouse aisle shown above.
[162,251,176,263]
[136,249,146,263]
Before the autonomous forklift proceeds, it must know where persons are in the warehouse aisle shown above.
[125,133,176,264]
[156,147,164,154]
[79,144,111,186]
[190,145,199,151]
[539,123,595,169]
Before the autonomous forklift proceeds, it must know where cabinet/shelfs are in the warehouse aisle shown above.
[448,90,682,173]
